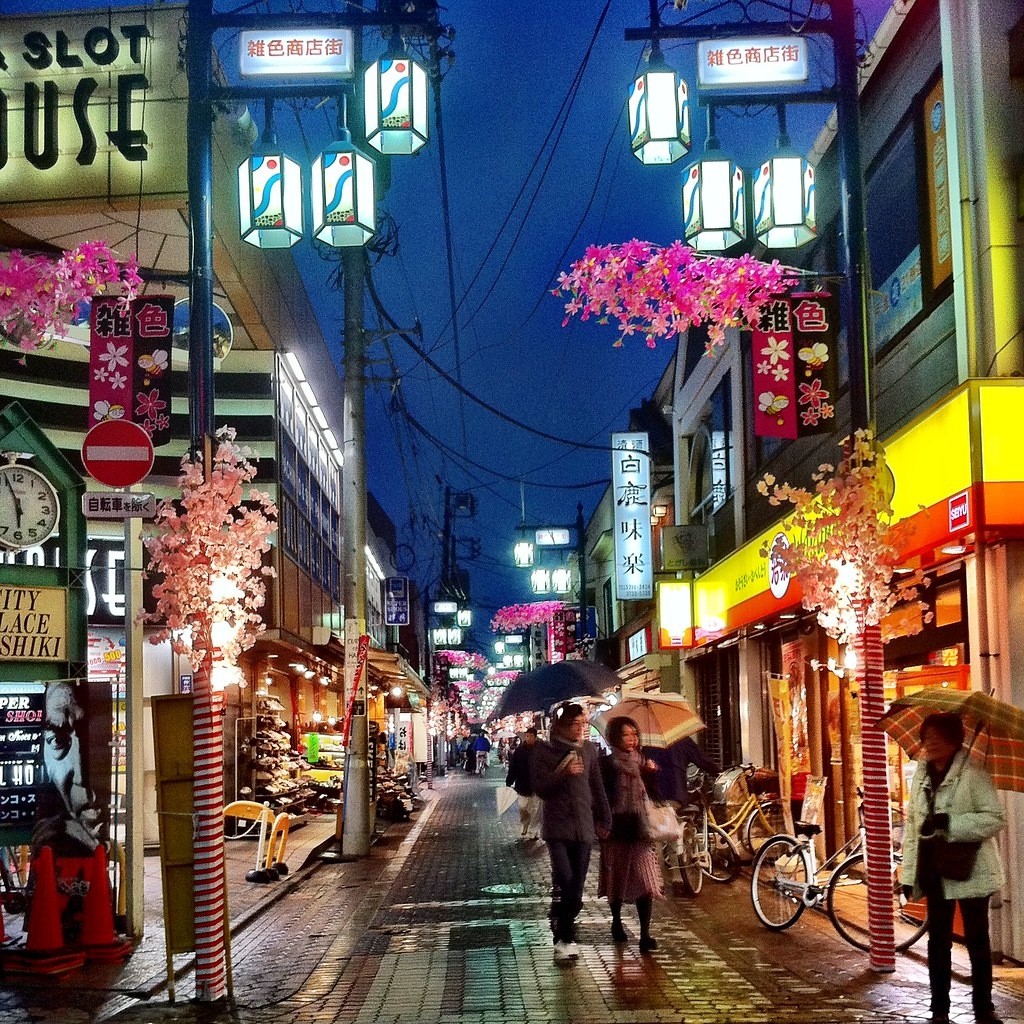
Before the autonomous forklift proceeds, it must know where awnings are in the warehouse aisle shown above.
[342,648,433,700]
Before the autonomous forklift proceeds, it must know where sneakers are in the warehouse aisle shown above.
[555,938,579,960]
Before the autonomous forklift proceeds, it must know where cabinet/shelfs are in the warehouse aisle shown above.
[299,728,345,803]
[249,693,311,837]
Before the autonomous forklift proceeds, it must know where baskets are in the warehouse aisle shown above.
[892,808,905,852]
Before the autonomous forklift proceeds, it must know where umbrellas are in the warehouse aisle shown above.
[585,682,707,765]
[495,728,517,738]
[470,729,489,738]
[486,659,628,761]
[495,783,519,816]
[873,688,1024,839]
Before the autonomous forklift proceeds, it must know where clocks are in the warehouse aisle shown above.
[0,464,61,550]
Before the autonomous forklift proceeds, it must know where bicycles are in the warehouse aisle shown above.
[477,754,486,777]
[673,764,787,895]
[751,786,931,955]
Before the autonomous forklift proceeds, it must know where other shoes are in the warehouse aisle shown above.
[639,938,657,950]
[975,1010,1003,1024]
[527,832,539,840]
[612,923,628,943]
[521,818,532,835]
[931,1014,949,1024]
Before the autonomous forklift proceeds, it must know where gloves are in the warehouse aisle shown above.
[903,885,913,901]
[920,812,949,836]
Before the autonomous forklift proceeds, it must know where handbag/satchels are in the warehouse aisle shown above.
[646,804,680,842]
[462,759,471,771]
[933,841,981,881]
[608,811,641,844]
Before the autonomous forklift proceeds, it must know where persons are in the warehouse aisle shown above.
[642,735,724,899]
[598,716,681,950]
[531,702,612,961]
[506,729,545,840]
[450,731,527,774]
[898,711,1006,1024]
[33,684,112,852]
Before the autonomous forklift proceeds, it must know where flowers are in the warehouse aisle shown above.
[757,425,935,714]
[489,600,608,640]
[0,241,146,367]
[549,236,885,357]
[135,423,278,731]
[433,652,519,725]
